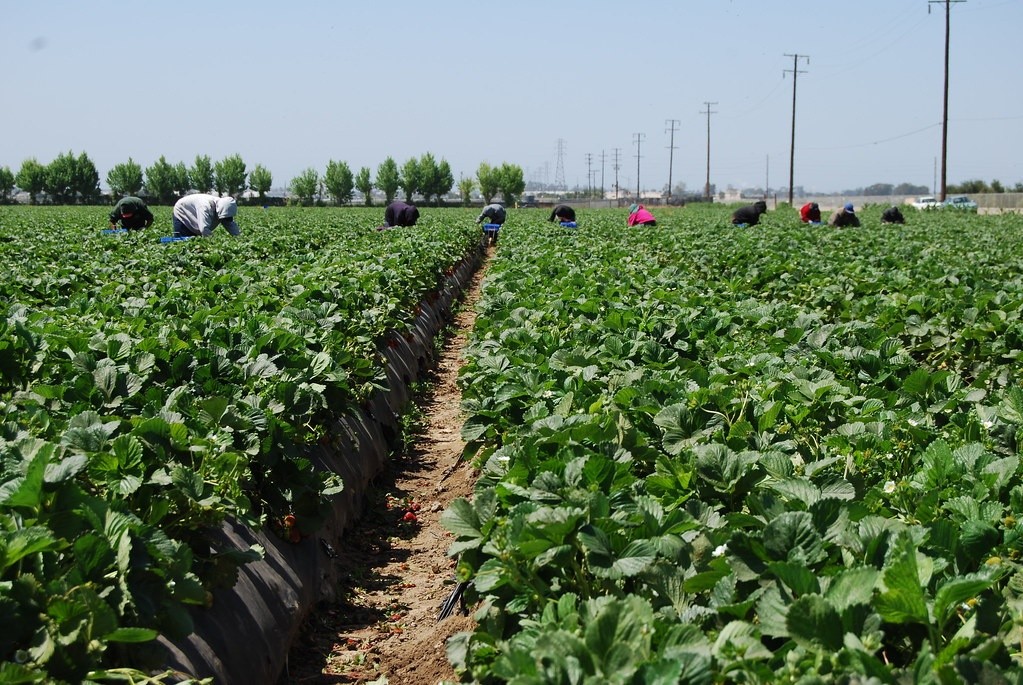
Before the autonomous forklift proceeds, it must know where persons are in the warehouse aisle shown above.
[475,204,506,247]
[627,204,656,227]
[547,205,575,225]
[799,203,821,226]
[731,201,767,229]
[880,206,905,224]
[172,193,240,238]
[828,203,861,228]
[385,201,420,228]
[109,196,154,231]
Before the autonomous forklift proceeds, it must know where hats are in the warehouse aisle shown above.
[812,203,818,211]
[844,203,854,214]
[627,204,639,214]
[756,200,767,214]
[889,206,898,217]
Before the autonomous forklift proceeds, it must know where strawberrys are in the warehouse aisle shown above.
[265,268,454,647]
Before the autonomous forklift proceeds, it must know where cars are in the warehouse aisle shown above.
[912,197,936,211]
[947,196,979,213]
